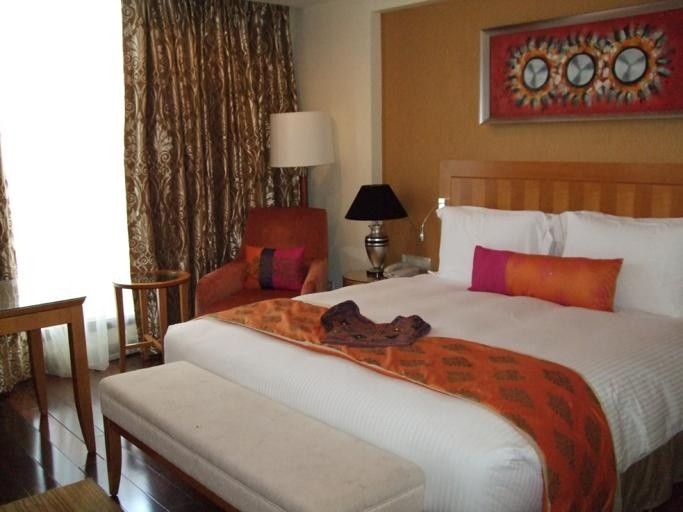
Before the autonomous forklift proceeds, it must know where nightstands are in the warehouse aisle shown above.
[343,269,388,286]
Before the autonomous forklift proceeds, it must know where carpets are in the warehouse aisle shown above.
[26,479,124,512]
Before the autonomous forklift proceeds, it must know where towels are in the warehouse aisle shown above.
[320,299,431,348]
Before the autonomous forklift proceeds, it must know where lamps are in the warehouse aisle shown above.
[344,183,408,275]
[269,110,335,208]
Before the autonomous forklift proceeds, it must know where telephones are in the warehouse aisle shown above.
[382,262,420,278]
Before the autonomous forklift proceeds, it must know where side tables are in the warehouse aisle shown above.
[112,267,191,373]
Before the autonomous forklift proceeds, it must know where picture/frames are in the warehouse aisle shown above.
[478,0,683,128]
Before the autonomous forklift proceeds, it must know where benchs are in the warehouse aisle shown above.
[97,360,428,512]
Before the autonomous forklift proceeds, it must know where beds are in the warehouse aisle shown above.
[160,161,683,512]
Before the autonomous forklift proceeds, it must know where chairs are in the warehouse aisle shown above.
[194,207,329,317]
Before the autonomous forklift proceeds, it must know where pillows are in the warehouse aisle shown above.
[468,245,624,312]
[553,210,683,321]
[243,243,307,290]
[428,205,560,285]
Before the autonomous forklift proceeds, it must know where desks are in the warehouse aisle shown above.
[0,276,96,453]
